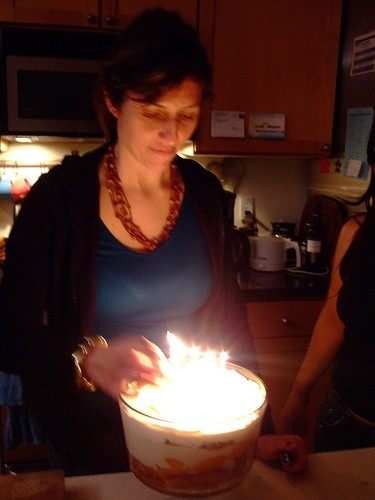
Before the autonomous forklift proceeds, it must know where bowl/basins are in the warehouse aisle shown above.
[113,357,267,497]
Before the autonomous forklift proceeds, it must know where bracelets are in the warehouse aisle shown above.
[72,334,110,392]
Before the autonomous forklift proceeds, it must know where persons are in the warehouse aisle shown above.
[278,116,375,456]
[0,6,307,477]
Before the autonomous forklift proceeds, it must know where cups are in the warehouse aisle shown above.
[0,167,33,201]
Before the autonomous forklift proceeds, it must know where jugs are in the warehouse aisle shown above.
[250,235,302,272]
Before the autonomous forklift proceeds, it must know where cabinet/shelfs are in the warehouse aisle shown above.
[0,0,342,157]
[243,299,330,435]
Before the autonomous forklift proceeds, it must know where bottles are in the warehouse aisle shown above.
[304,202,324,270]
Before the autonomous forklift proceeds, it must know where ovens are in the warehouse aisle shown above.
[6,53,113,136]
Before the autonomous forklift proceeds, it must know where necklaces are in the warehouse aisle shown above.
[104,143,183,249]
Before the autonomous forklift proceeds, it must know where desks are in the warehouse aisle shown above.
[65,448,375,500]
